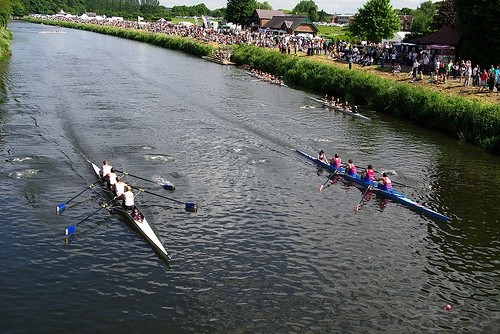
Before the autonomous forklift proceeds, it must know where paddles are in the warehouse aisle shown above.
[390,180,426,191]
[115,169,175,190]
[64,197,117,236]
[57,177,103,213]
[355,184,371,211]
[341,162,379,173]
[318,167,342,191]
[129,186,198,210]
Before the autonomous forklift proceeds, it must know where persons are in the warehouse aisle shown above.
[106,168,117,188]
[331,153,342,167]
[378,173,392,189]
[131,208,141,220]
[144,21,331,56]
[343,159,356,175]
[34,16,72,21]
[361,165,375,181]
[72,16,146,29]
[115,186,134,210]
[318,149,326,161]
[328,38,408,73]
[100,160,113,178]
[408,50,500,94]
[111,176,127,197]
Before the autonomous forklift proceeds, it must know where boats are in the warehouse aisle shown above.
[305,94,372,122]
[300,105,316,109]
[91,162,172,261]
[200,55,236,65]
[246,71,288,87]
[296,148,453,221]
